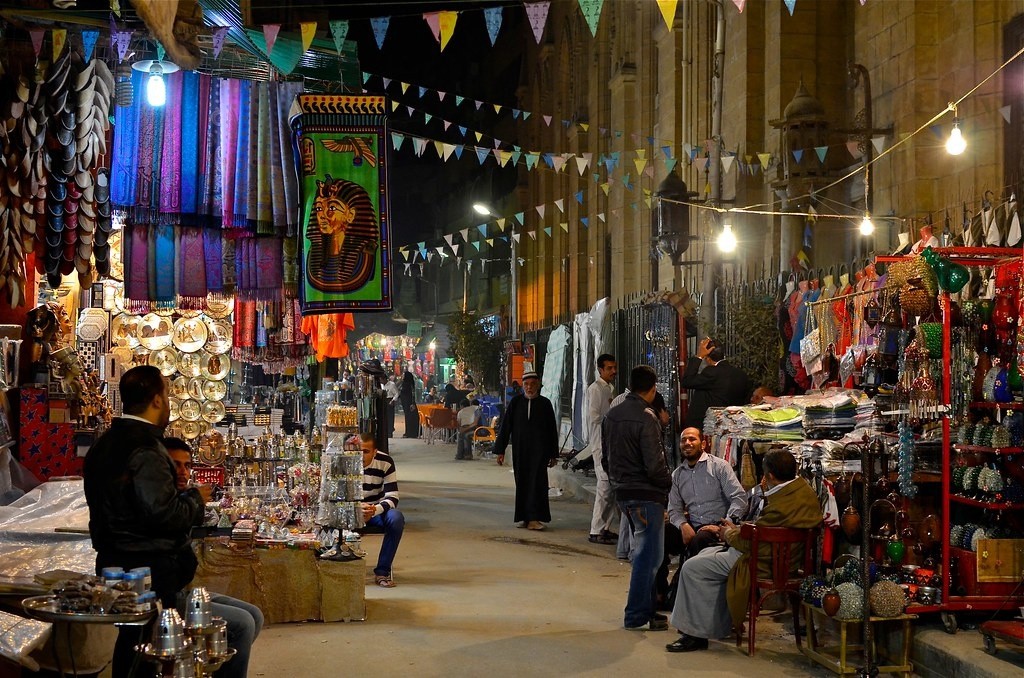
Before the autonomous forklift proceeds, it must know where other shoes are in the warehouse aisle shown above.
[515,522,526,527]
[527,522,547,530]
[652,613,668,621]
[625,620,668,630]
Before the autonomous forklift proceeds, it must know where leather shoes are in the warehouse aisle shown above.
[667,634,708,652]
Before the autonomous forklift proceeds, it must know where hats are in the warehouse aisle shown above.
[521,371,538,380]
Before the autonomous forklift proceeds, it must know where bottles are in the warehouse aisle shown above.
[103,565,155,613]
[974,297,1023,403]
[224,421,321,487]
[155,585,227,678]
[920,245,970,294]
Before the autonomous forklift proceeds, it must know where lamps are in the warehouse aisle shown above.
[131,36,180,112]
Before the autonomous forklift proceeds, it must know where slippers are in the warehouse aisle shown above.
[376,578,397,588]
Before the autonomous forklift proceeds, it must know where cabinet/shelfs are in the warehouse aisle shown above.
[943,401,1024,611]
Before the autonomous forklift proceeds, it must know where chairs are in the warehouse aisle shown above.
[736,523,820,658]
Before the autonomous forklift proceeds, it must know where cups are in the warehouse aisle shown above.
[882,563,942,605]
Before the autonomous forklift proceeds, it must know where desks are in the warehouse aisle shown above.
[801,600,919,677]
[205,544,367,625]
[417,404,454,445]
[698,433,944,566]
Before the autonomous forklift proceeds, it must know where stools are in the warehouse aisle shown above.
[359,534,394,581]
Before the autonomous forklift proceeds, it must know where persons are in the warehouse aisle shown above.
[342,369,351,382]
[751,387,772,404]
[678,338,750,434]
[29,301,81,388]
[164,437,263,678]
[444,384,472,407]
[667,426,749,553]
[600,365,673,631]
[424,386,439,403]
[397,372,422,438]
[454,398,488,460]
[379,374,399,437]
[353,433,405,587]
[778,224,939,389]
[492,372,559,530]
[584,354,620,544]
[82,366,212,678]
[666,450,822,651]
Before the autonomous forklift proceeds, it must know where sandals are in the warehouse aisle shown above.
[588,535,612,545]
[601,530,619,538]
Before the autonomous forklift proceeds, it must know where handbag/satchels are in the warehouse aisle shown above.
[798,294,885,387]
[739,441,757,490]
